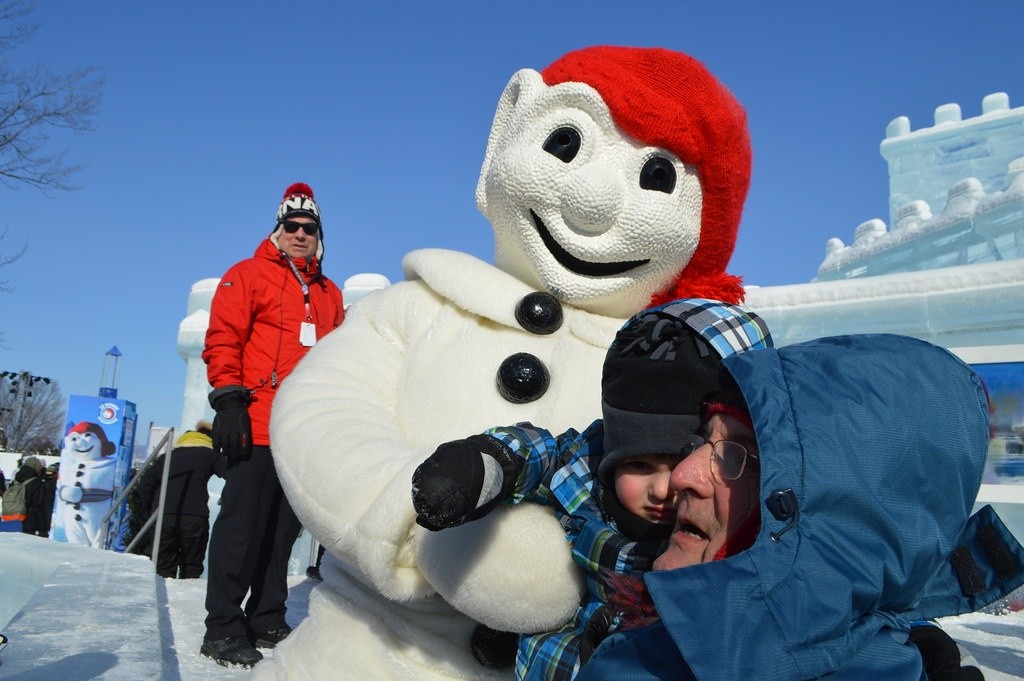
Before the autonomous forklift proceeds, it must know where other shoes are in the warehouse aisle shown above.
[305,565,324,581]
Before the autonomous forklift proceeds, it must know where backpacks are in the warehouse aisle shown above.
[0,477,37,521]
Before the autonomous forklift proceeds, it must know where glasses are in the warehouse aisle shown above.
[280,220,318,236]
[682,433,760,481]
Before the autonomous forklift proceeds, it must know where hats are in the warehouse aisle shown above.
[599,317,737,555]
[273,182,325,275]
[45,465,58,473]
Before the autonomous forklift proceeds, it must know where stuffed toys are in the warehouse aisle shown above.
[242,45,753,681]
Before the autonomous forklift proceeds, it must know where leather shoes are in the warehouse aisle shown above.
[258,620,292,649]
[200,635,263,670]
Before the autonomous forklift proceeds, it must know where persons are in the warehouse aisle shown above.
[412,298,774,681]
[572,335,1023,681]
[199,183,345,668]
[0,457,61,538]
[134,421,224,580]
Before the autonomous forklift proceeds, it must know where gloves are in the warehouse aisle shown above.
[410,432,520,531]
[209,386,254,469]
